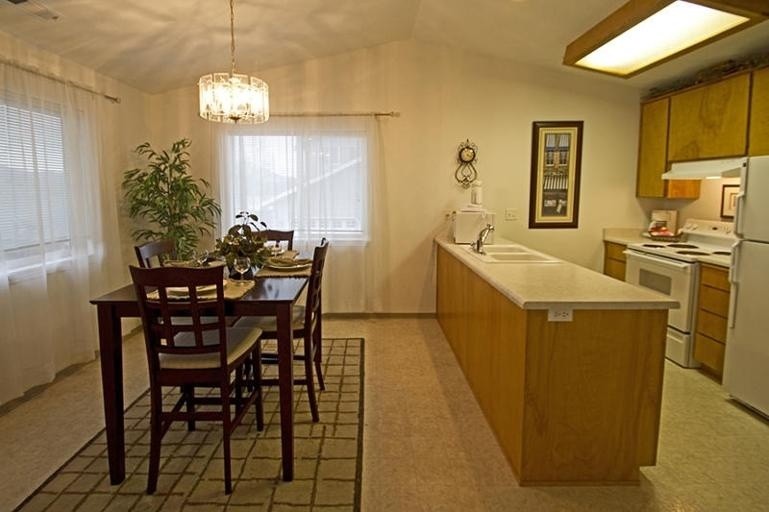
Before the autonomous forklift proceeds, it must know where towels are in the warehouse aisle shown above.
[163,256,213,267]
[267,256,313,267]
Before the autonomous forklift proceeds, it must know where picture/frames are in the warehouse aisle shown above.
[721,184,740,218]
[528,120,584,229]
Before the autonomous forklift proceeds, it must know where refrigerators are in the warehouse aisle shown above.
[721,154,769,423]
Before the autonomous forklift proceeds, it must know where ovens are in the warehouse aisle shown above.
[622,249,701,369]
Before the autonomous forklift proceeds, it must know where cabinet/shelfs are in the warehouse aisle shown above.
[747,66,769,157]
[666,70,753,163]
[433,237,680,486]
[636,95,700,199]
[604,240,627,283]
[694,258,730,384]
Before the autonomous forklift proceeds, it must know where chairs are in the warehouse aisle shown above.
[252,229,294,256]
[134,237,240,394]
[129,265,264,496]
[232,238,331,423]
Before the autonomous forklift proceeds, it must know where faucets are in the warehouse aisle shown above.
[470,224,495,255]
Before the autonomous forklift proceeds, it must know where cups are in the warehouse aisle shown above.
[234,256,251,280]
[193,248,208,266]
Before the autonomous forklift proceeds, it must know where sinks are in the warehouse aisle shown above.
[471,252,562,264]
[460,244,538,252]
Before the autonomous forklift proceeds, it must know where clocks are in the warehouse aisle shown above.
[455,139,480,189]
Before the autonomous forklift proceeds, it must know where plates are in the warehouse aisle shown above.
[166,279,227,295]
[267,265,309,271]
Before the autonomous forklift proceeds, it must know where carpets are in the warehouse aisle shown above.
[14,338,364,512]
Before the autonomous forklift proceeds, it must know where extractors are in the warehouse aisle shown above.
[661,157,741,180]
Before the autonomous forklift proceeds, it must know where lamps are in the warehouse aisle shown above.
[198,0,268,125]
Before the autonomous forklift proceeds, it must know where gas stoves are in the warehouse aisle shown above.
[628,218,734,268]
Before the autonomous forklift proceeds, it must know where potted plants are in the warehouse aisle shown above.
[214,212,272,279]
